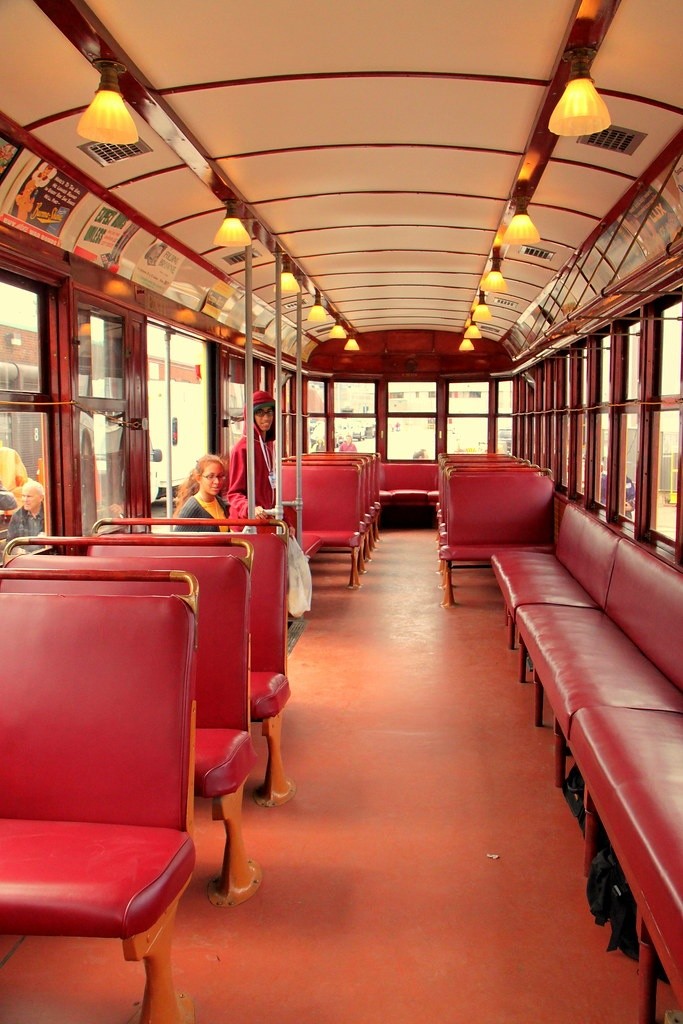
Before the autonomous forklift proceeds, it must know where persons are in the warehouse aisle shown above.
[602,472,635,519]
[312,438,326,451]
[413,449,429,459]
[227,390,296,536]
[6,481,45,555]
[339,435,358,452]
[174,455,230,532]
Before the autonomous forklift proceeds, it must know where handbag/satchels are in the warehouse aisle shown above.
[0,481,18,510]
[286,536,312,619]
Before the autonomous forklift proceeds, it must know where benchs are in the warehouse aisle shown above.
[0,517,291,1024]
[491,506,683,1024]
[276,451,555,608]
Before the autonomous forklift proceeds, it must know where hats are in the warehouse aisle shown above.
[254,402,274,412]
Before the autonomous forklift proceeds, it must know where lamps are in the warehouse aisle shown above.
[214,200,252,246]
[459,339,474,351]
[76,60,139,144]
[307,289,326,321]
[547,45,610,136]
[273,261,300,295]
[503,201,541,245]
[5,333,23,346]
[344,332,360,350]
[464,311,483,339]
[329,317,347,338]
[471,291,493,321]
[481,247,509,293]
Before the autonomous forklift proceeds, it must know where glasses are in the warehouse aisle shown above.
[255,409,274,417]
[200,473,226,482]
